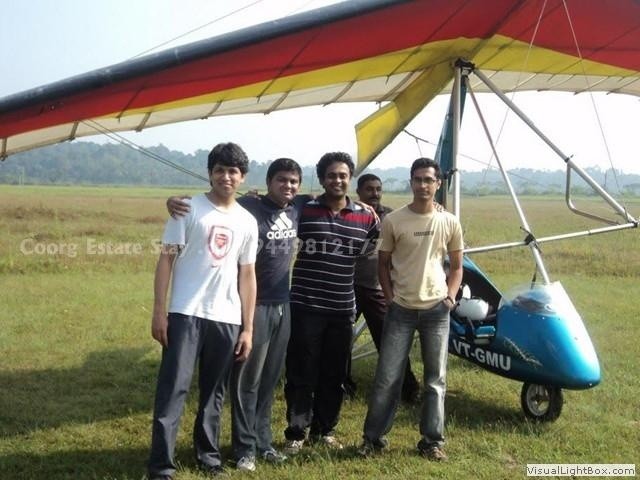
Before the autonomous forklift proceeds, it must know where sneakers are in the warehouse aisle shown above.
[287,438,306,454]
[320,436,343,452]
[417,440,448,463]
[236,456,256,475]
[207,467,231,478]
[357,439,390,458]
[262,449,285,465]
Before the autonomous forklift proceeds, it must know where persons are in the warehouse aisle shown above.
[148,142,259,480]
[167,158,380,472]
[357,158,464,462]
[342,173,420,403]
[247,152,447,456]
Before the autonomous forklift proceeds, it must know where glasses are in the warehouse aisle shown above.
[412,177,439,184]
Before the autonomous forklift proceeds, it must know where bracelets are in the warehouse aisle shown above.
[447,295,457,305]
